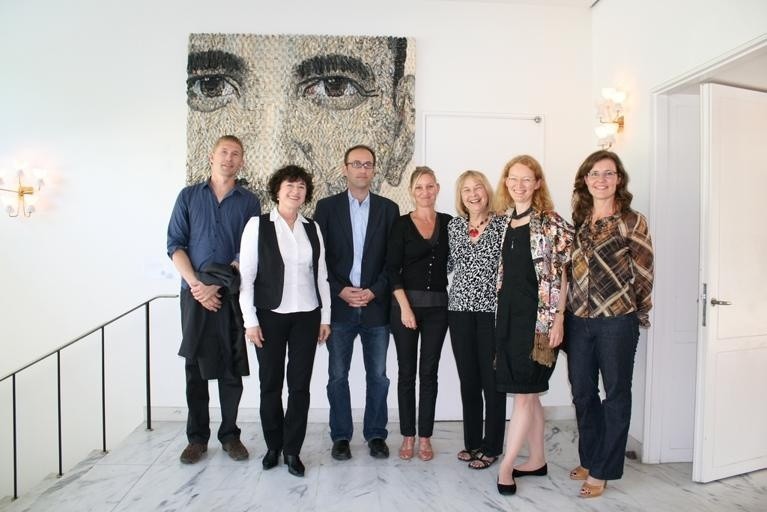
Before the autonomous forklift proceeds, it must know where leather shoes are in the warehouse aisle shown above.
[262,449,281,470]
[179,443,208,464]
[368,438,389,459]
[222,440,249,461]
[284,454,306,477]
[331,440,352,461]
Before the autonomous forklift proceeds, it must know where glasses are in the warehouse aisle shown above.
[589,171,618,178]
[347,160,373,169]
[506,176,535,184]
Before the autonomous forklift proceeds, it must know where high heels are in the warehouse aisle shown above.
[578,478,608,498]
[570,464,590,480]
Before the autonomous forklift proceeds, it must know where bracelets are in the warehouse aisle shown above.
[554,309,565,315]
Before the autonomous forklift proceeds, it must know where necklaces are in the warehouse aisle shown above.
[512,207,532,219]
[468,213,490,237]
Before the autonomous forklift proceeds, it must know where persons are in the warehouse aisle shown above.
[239,165,333,477]
[188,33,417,220]
[314,144,405,460]
[562,150,654,498]
[166,134,262,464]
[492,156,575,495]
[386,166,455,461]
[445,170,509,469]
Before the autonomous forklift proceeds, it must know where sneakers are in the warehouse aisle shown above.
[496,471,517,496]
[513,463,548,478]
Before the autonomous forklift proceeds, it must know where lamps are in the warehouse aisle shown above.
[598,84,624,127]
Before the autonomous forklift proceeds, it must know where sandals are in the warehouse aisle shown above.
[468,452,499,470]
[418,437,433,461]
[399,436,416,460]
[457,448,483,462]
